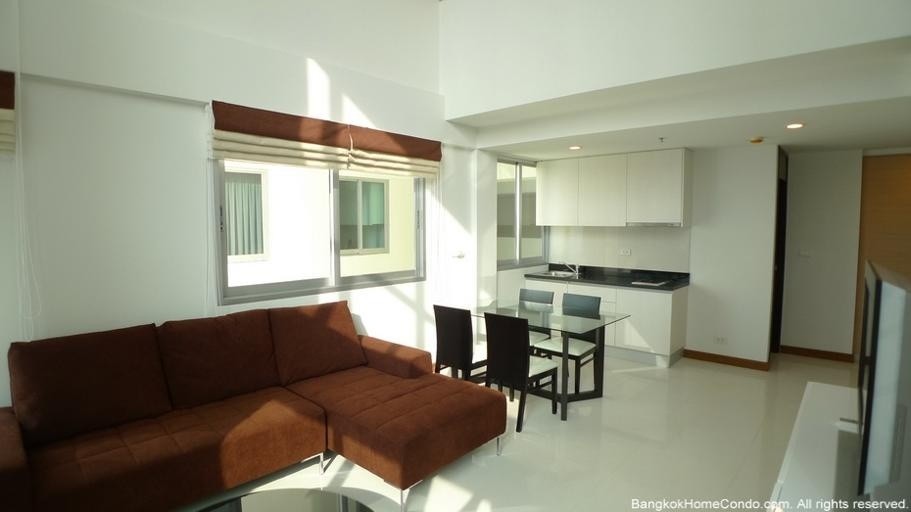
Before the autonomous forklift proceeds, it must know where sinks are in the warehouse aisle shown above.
[525,269,582,278]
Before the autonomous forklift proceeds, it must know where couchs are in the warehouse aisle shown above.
[0,300,505,512]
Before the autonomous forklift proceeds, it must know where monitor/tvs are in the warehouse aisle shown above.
[856,258,911,496]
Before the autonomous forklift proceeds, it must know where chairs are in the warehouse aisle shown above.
[434,287,605,432]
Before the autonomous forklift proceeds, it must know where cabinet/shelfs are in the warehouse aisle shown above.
[534,147,694,230]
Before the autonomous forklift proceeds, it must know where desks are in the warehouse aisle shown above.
[470,301,632,403]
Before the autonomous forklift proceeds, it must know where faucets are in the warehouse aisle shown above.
[561,261,580,273]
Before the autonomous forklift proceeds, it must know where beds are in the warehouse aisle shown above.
[198,487,375,512]
